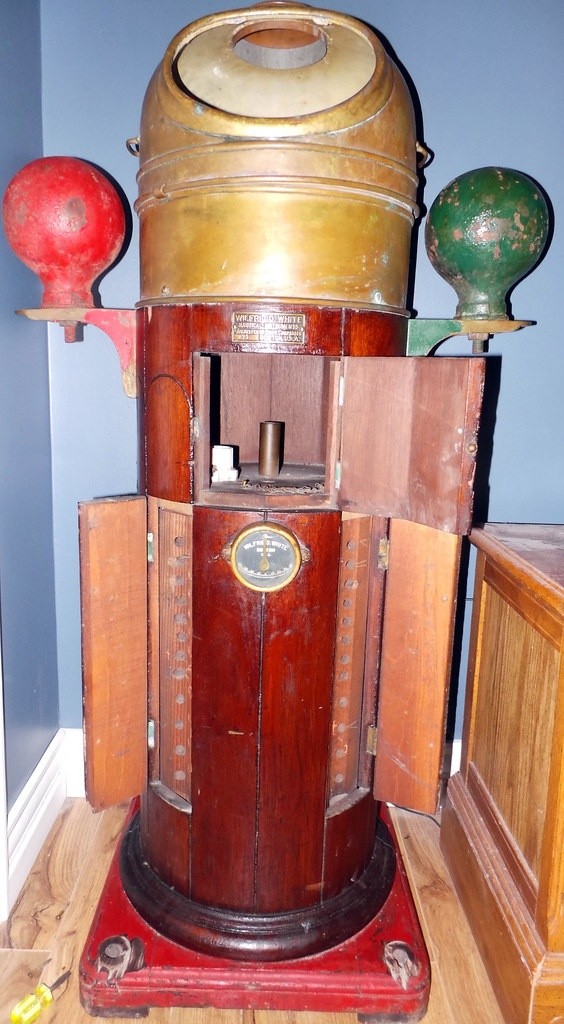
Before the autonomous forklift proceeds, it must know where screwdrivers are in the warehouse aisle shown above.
[11,970,73,1024]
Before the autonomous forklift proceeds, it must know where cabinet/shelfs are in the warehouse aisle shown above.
[440,523,564,1024]
